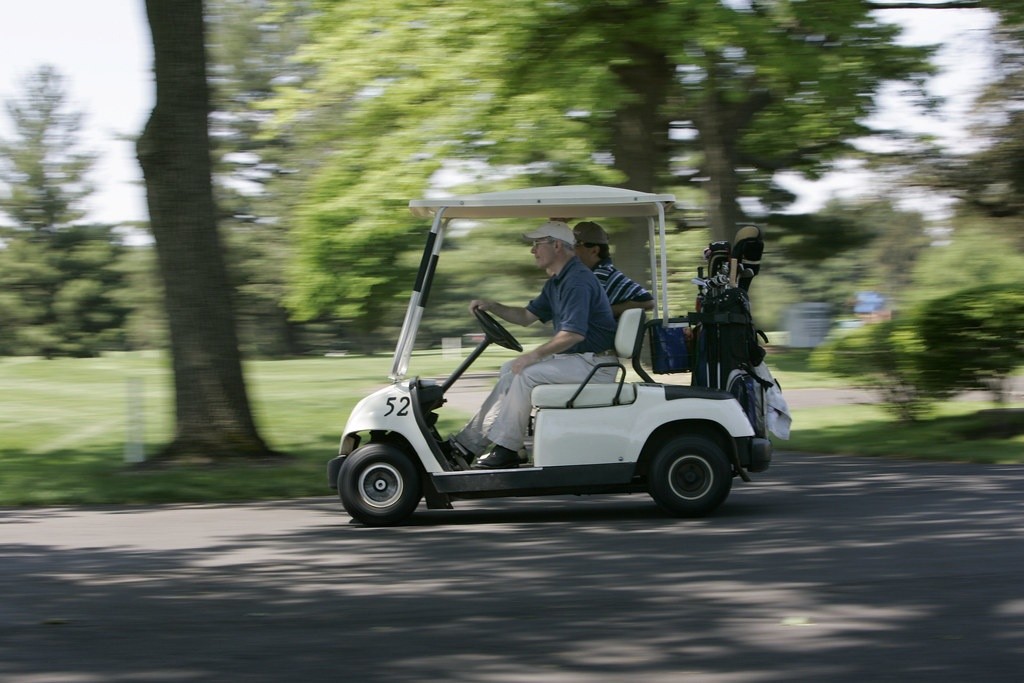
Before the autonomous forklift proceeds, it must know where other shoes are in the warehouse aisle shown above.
[474,445,518,467]
[435,439,474,466]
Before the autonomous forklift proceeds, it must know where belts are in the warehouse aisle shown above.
[596,351,615,356]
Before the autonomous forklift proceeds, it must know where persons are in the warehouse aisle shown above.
[436,221,618,468]
[478,221,654,463]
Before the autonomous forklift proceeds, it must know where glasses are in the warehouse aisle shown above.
[575,242,582,248]
[533,241,552,246]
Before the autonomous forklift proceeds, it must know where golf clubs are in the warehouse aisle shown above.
[689,225,765,390]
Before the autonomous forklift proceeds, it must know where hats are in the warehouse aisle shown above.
[574,221,609,243]
[522,221,576,246]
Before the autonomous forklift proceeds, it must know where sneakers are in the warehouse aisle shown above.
[518,448,528,463]
